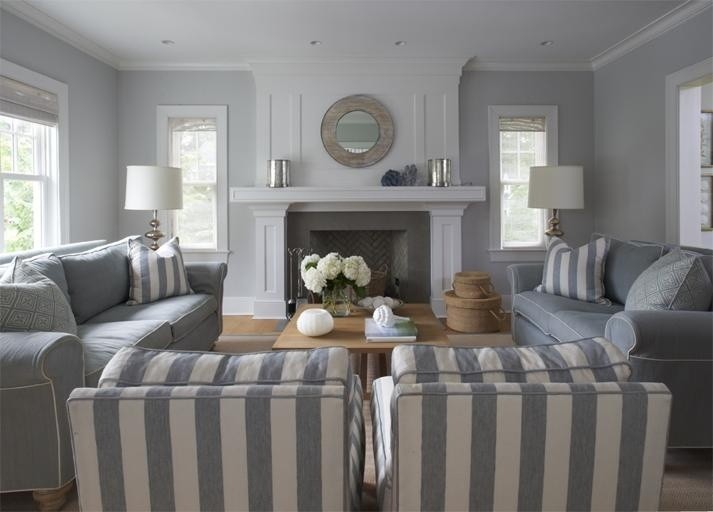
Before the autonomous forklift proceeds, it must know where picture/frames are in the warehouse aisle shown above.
[701,109,713,168]
[701,173,713,231]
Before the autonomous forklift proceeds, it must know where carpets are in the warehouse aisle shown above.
[59,334,713,511]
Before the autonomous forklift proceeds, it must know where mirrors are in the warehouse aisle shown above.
[321,96,394,168]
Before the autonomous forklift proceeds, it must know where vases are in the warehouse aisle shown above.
[322,285,353,317]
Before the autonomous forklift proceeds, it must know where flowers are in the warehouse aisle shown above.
[301,252,371,315]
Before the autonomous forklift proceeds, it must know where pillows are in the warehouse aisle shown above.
[58,235,143,325]
[126,237,194,306]
[533,234,612,308]
[0,253,71,304]
[625,251,713,311]
[0,256,77,336]
[591,233,664,305]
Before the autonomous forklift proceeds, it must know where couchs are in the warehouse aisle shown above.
[371,337,673,512]
[0,236,227,512]
[507,232,713,449]
[65,346,365,512]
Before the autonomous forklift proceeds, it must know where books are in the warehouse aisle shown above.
[365,317,418,342]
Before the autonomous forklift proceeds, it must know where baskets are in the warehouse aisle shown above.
[364,264,388,296]
[442,290,507,333]
[452,272,495,299]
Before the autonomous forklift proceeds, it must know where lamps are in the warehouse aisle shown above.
[528,166,584,243]
[125,166,183,251]
